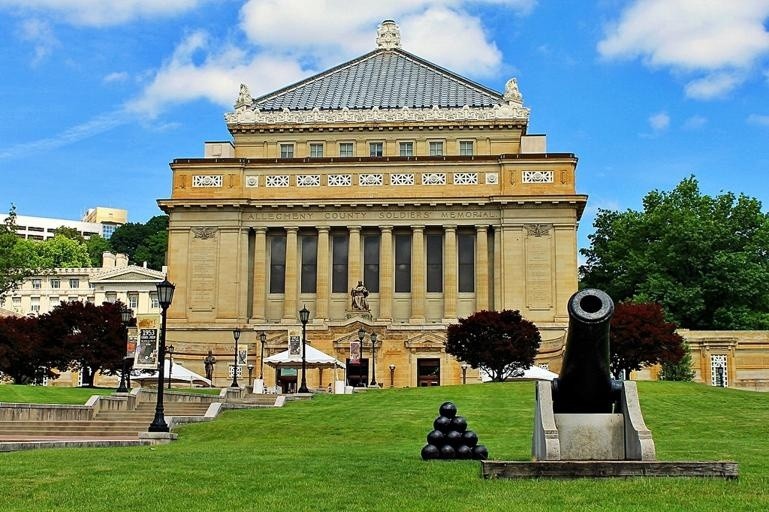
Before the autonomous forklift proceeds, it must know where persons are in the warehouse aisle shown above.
[203,351,216,381]
[143,339,154,360]
[351,279,369,311]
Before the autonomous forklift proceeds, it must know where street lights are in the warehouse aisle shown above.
[147,272,176,431]
[318,362,324,389]
[357,325,366,387]
[231,326,240,387]
[389,364,396,387]
[247,365,254,386]
[718,364,724,387]
[259,331,266,379]
[168,343,174,388]
[460,364,468,384]
[116,305,132,393]
[370,330,377,385]
[299,304,310,393]
